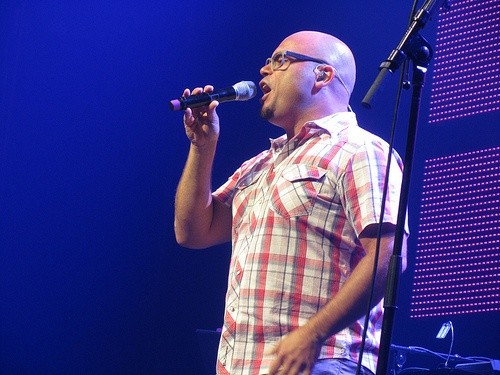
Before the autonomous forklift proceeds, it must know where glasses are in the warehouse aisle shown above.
[265,50,329,70]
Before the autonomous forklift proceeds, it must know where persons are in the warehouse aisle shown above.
[173,31,411,375]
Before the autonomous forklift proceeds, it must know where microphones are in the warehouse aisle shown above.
[169,80,257,111]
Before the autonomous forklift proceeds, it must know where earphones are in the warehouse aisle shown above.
[313,65,327,80]
